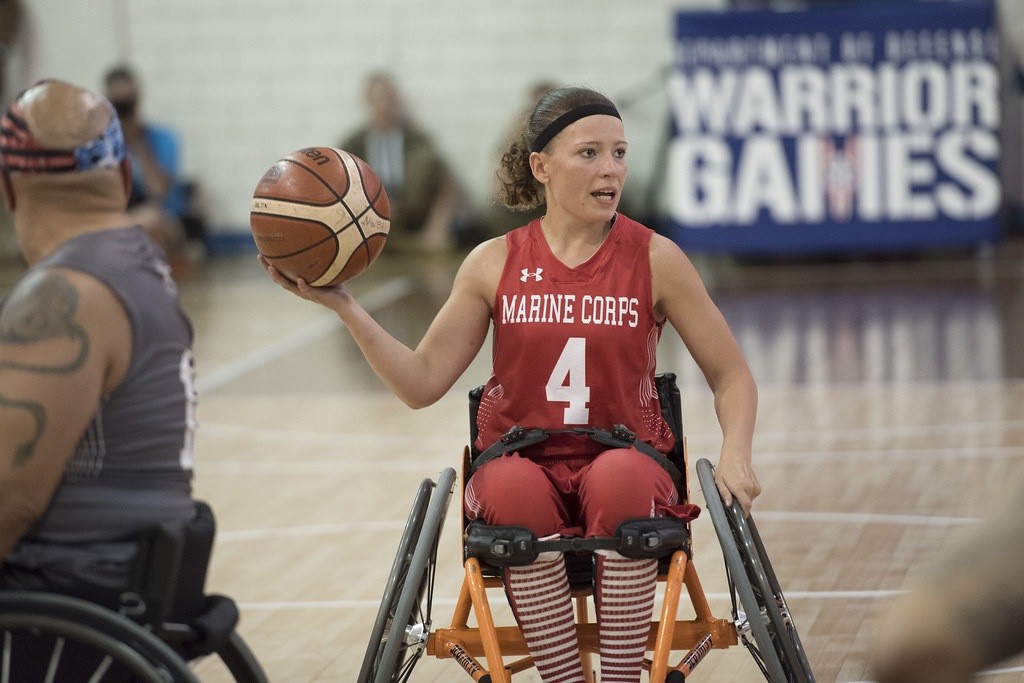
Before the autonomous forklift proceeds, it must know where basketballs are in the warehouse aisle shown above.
[248,146,391,290]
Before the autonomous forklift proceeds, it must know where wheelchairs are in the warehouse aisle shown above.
[0,499,275,682]
[354,371,816,683]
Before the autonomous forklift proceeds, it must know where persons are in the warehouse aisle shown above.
[99,67,455,253]
[0,77,196,683]
[258,85,760,683]
